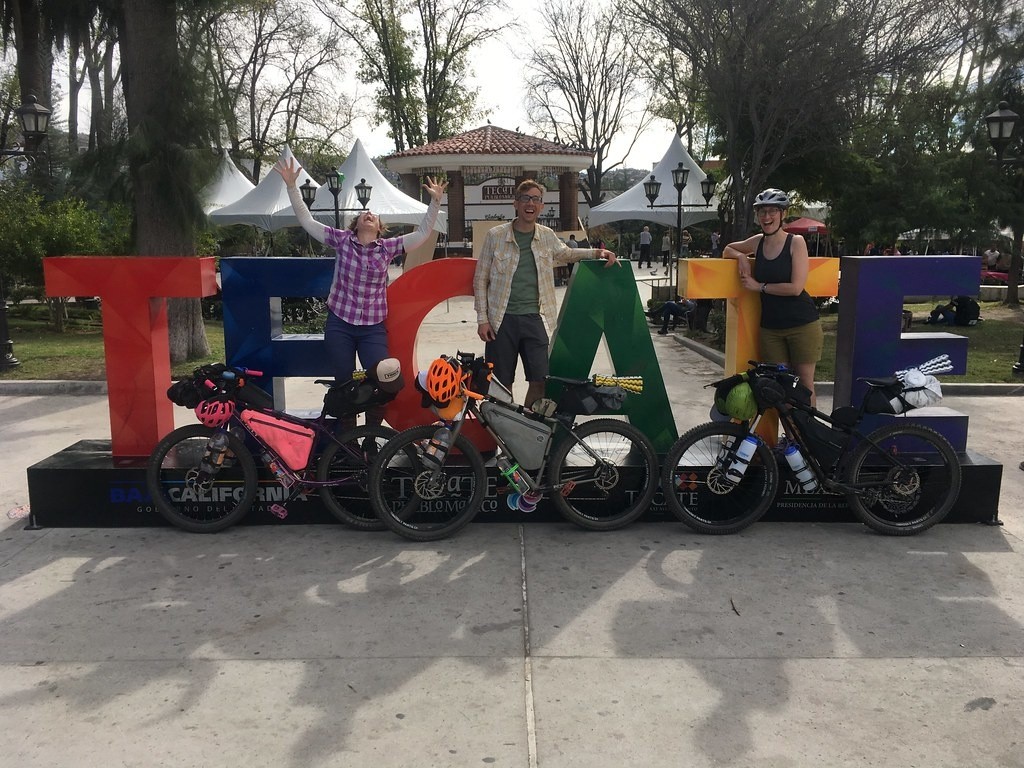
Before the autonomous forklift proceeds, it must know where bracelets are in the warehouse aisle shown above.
[762,283,767,294]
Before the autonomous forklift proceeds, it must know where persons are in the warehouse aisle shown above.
[711,230,721,257]
[682,230,692,258]
[638,225,652,268]
[802,234,847,257]
[985,245,1000,271]
[272,156,450,469]
[723,187,823,444]
[565,234,607,278]
[662,230,671,267]
[473,179,622,416]
[928,296,980,326]
[864,239,960,256]
[644,299,697,334]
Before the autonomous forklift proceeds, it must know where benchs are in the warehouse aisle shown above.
[669,299,714,332]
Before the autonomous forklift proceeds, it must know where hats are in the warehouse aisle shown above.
[366,357,404,393]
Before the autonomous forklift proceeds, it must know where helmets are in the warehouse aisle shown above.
[427,357,461,404]
[753,187,789,206]
[196,396,232,427]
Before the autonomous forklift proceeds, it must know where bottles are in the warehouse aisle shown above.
[495,454,529,494]
[419,418,447,455]
[199,427,230,475]
[259,447,293,487]
[726,436,758,483]
[784,446,818,490]
[221,423,246,468]
[716,435,736,470]
[421,424,453,470]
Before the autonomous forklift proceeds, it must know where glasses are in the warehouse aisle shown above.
[517,195,542,204]
[758,208,782,216]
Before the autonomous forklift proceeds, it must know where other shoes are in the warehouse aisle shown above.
[658,330,668,334]
[638,266,642,268]
[643,312,661,319]
[647,266,652,268]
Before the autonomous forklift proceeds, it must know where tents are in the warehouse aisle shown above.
[897,217,1024,257]
[195,147,259,232]
[206,142,322,257]
[585,132,725,257]
[272,138,450,312]
[783,216,831,257]
[719,152,832,224]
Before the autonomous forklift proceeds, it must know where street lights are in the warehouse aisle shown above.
[642,162,718,329]
[983,100,1024,374]
[0,94,54,371]
[298,164,374,229]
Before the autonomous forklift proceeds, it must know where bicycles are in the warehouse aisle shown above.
[145,361,424,534]
[365,349,661,542]
[660,360,963,537]
